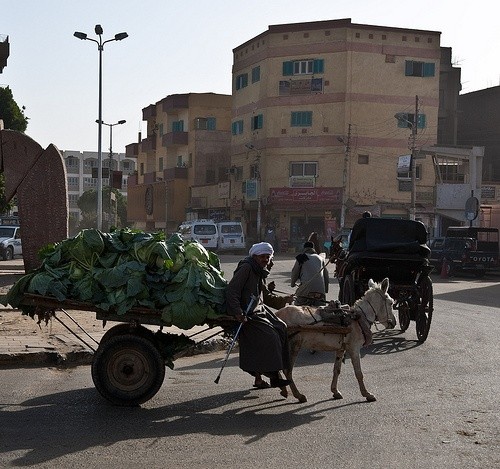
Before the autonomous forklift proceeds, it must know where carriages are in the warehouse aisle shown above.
[329,217,434,341]
[17,276,397,408]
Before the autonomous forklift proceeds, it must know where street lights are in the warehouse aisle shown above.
[336,123,352,228]
[95,118,126,226]
[73,24,129,232]
[393,94,420,222]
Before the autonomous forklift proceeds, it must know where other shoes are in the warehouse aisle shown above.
[269,377,291,389]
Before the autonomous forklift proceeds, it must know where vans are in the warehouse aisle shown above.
[177,218,246,253]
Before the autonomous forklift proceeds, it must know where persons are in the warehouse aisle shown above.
[292,242,329,308]
[225,241,291,388]
[348,211,371,246]
[249,251,298,387]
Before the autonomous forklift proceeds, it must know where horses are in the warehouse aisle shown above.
[329,236,349,286]
[273,277,397,403]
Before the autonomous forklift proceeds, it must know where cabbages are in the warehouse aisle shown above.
[0,227,231,329]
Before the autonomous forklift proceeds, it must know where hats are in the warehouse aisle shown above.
[249,241,274,257]
[304,241,314,248]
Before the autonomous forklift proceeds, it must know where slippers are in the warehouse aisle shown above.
[252,380,272,389]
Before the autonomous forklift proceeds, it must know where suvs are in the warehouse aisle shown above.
[0,224,23,260]
[444,225,500,279]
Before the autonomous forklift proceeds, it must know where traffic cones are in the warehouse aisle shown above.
[440,257,448,279]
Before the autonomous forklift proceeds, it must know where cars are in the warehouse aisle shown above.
[429,237,445,268]
